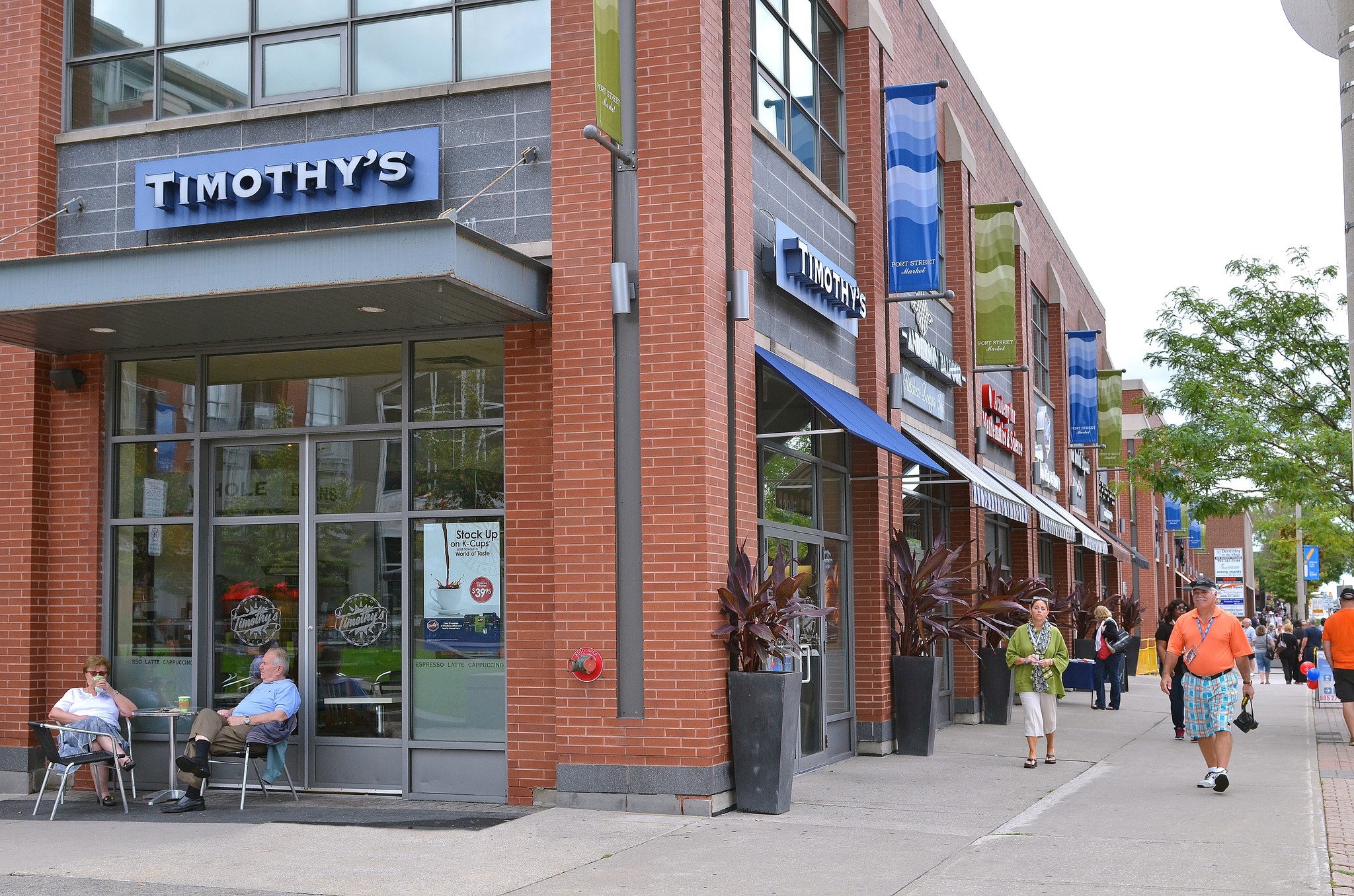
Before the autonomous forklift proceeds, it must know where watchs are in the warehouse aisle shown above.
[112,690,119,698]
[1243,680,1253,686]
[244,716,250,725]
[158,688,163,693]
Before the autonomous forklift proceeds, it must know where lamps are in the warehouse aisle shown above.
[1068,486,1077,505]
[726,269,749,323]
[1118,518,1125,533]
[1030,462,1041,485]
[1175,558,1198,579]
[1166,553,1170,564]
[1096,504,1105,522]
[974,426,987,454]
[1156,547,1160,559]
[609,261,637,317]
[887,373,903,410]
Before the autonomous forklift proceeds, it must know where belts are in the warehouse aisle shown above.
[1188,668,1232,681]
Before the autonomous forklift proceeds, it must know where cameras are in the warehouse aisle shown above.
[1233,712,1259,733]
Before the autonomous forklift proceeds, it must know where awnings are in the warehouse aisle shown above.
[755,345,1195,584]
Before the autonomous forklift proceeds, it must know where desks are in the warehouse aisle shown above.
[130,706,200,806]
[323,692,402,737]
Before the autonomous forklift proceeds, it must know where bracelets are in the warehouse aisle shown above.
[1024,658,1028,664]
[1048,658,1053,666]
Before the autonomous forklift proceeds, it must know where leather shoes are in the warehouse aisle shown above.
[175,755,211,779]
[160,795,206,812]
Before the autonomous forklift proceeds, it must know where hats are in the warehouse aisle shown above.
[1340,588,1354,600]
[1189,577,1217,592]
[1269,611,1275,614]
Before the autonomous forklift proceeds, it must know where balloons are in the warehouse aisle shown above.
[1306,668,1320,681]
[1306,679,1318,690]
[1300,662,1314,675]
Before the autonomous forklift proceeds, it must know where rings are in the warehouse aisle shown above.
[1043,665,1044,667]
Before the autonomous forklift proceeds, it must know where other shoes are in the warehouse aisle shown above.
[1260,682,1263,684]
[121,759,136,771]
[1091,705,1104,710]
[1266,682,1270,684]
[1250,672,1254,676]
[97,794,117,806]
[1348,737,1354,746]
[1106,706,1119,710]
[1286,681,1303,685]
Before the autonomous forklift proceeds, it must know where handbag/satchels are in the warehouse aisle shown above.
[1104,620,1134,654]
[1275,633,1287,654]
[1265,649,1275,660]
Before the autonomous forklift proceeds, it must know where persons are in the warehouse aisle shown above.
[118,654,193,735]
[248,639,292,679]
[161,647,301,813]
[48,655,139,806]
[1007,576,1353,794]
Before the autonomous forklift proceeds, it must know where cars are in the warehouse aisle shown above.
[1332,605,1341,614]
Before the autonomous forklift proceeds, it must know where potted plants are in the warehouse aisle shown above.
[976,547,1147,725]
[882,527,1032,757]
[710,535,836,817]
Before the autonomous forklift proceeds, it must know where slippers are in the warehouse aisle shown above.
[1045,754,1055,764]
[1024,758,1037,769]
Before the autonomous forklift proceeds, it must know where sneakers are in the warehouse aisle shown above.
[1213,769,1229,792]
[1174,727,1185,740]
[1197,771,1217,788]
[1190,736,1198,742]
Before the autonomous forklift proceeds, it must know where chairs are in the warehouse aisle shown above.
[318,670,402,737]
[27,717,135,821]
[200,715,299,809]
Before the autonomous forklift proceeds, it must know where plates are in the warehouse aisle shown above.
[427,601,472,615]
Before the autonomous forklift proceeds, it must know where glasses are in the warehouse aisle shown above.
[86,671,108,676]
[1176,605,1188,611]
[1190,581,1216,587]
[1031,596,1048,610]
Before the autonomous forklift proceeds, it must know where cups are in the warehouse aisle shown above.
[483,628,487,634]
[178,696,191,712]
[92,675,105,692]
[429,586,463,610]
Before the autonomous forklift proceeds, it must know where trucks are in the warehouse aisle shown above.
[1308,591,1334,621]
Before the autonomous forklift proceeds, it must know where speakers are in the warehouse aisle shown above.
[50,368,82,391]
